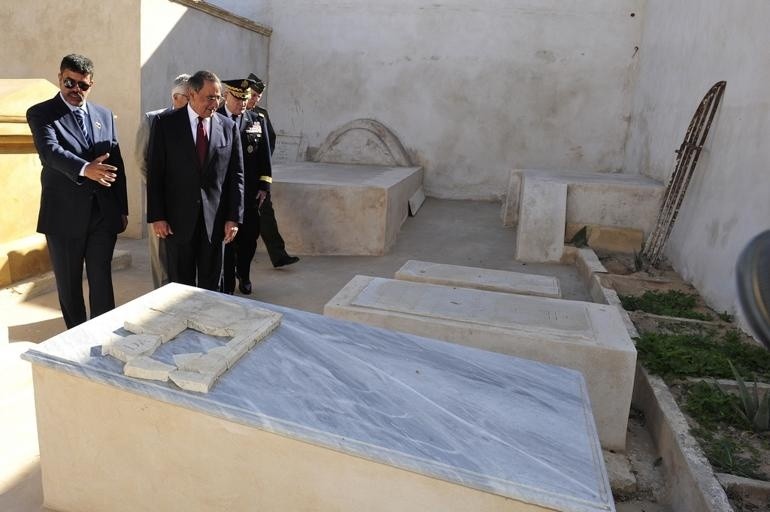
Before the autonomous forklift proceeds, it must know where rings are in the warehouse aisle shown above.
[231,227,234,231]
[156,232,160,236]
[234,226,238,231]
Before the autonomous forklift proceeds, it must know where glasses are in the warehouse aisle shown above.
[62,76,90,91]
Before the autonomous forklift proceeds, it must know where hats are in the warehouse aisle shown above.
[247,73,264,94]
[221,78,256,101]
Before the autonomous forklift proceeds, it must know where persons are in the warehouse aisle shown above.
[245,71,299,269]
[211,78,273,295]
[26,54,129,329]
[147,70,244,291]
[134,72,192,289]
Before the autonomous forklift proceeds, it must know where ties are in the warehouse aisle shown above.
[231,114,237,121]
[72,109,94,148]
[195,116,208,159]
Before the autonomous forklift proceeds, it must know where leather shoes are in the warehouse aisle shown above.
[274,256,299,267]
[236,272,251,294]
